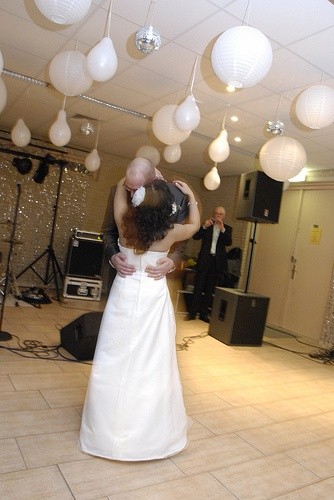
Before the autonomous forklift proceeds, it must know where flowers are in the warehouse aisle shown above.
[131,186,146,207]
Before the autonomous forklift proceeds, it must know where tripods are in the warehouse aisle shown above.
[15,166,65,302]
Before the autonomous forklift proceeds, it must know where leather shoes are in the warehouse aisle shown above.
[199,315,210,322]
[184,314,196,320]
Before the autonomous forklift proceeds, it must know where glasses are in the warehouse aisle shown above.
[122,177,138,192]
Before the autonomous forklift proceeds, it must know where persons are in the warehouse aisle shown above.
[99,157,190,307]
[77,168,202,463]
[183,206,233,323]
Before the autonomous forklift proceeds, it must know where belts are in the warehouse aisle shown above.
[209,254,216,257]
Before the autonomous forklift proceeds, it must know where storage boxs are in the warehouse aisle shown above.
[62,275,103,302]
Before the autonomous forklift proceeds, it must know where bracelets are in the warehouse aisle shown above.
[187,201,198,206]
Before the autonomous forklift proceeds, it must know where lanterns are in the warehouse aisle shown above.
[211,26,272,89]
[136,146,160,166]
[48,50,94,96]
[34,0,91,25]
[152,105,192,145]
[295,86,334,129]
[259,137,307,182]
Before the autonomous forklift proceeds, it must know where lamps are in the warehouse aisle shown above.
[259,136,308,183]
[10,118,32,148]
[80,122,95,136]
[204,162,221,191]
[175,52,201,133]
[48,94,72,147]
[208,103,230,163]
[52,38,94,97]
[151,98,191,145]
[33,0,93,26]
[295,68,334,130]
[264,94,286,135]
[11,155,33,175]
[83,119,102,173]
[210,0,275,93]
[164,144,182,163]
[133,0,162,56]
[85,0,118,83]
[32,159,50,184]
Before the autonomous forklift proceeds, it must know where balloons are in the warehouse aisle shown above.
[85,149,100,171]
[163,144,181,164]
[49,111,71,147]
[11,119,31,148]
[208,131,229,163]
[204,167,220,190]
[175,95,200,131]
[87,38,117,82]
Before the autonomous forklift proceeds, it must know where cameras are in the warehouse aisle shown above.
[210,217,216,223]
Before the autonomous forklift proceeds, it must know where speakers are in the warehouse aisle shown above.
[65,236,107,280]
[236,170,284,224]
[208,287,270,346]
